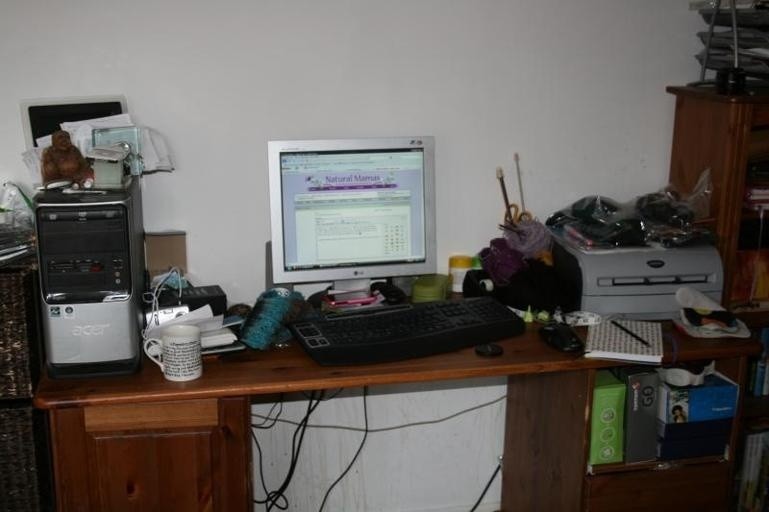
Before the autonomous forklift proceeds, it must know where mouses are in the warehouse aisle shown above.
[538,323,584,352]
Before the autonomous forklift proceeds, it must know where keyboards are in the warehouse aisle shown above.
[286,296,527,366]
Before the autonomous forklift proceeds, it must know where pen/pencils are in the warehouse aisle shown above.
[564,224,592,247]
[609,319,652,347]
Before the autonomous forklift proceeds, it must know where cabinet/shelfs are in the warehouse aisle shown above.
[665,86,769,512]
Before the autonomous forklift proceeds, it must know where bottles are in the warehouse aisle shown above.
[447,255,481,294]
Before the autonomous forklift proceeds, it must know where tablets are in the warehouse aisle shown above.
[20,94,128,150]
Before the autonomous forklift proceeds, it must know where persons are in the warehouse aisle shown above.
[41,130,88,188]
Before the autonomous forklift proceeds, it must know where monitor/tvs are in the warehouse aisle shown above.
[267,135,438,313]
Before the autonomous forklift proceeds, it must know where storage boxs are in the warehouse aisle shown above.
[587,365,741,465]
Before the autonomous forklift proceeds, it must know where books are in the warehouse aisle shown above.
[195,310,248,356]
[583,317,667,368]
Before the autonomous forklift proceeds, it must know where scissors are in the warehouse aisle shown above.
[504,204,532,225]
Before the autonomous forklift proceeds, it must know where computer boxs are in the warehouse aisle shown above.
[33,174,146,381]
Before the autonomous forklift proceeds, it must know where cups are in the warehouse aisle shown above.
[144,325,202,382]
[715,67,745,94]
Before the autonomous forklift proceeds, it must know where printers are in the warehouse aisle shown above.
[546,224,726,321]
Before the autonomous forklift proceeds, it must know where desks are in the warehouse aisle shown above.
[34,292,763,512]
